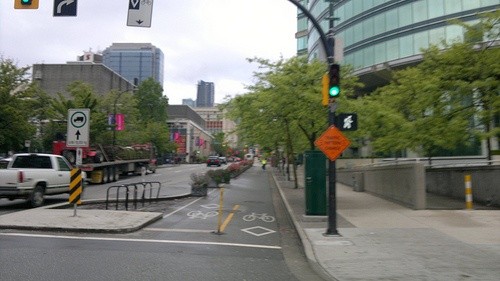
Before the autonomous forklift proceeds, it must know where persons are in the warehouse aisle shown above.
[262,158,267,170]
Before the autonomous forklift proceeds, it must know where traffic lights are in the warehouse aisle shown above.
[330,64,339,98]
[14,0,38,9]
[334,112,357,131]
[320,72,331,106]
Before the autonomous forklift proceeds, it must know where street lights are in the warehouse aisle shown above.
[111,88,141,146]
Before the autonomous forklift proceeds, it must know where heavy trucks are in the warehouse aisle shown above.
[52,138,153,187]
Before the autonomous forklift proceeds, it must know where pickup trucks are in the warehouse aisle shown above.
[0,152,87,209]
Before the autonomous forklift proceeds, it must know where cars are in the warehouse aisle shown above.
[207,155,221,166]
[227,155,241,164]
[219,157,226,165]
